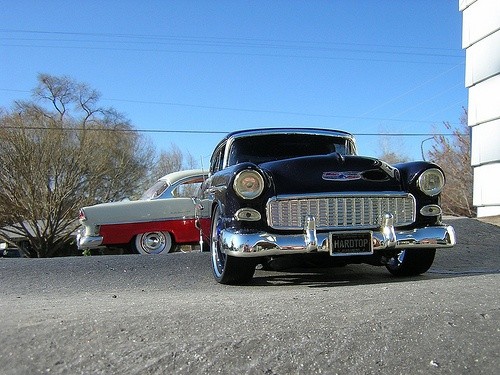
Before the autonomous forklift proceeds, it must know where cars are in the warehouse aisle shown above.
[192,126,456,287]
[76,170,219,257]
